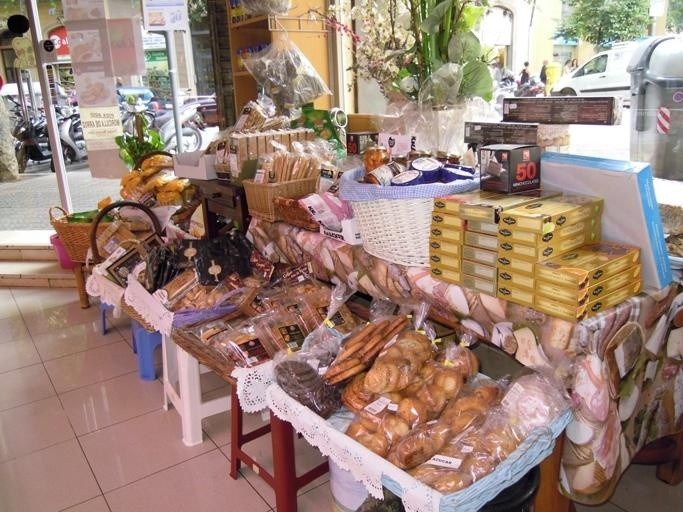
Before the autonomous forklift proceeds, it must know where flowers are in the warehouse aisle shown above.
[324,0,417,88]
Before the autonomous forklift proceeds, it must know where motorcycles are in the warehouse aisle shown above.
[6,95,202,174]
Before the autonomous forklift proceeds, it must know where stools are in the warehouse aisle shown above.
[160,332,271,447]
[99,300,136,354]
[76,267,92,309]
[228,382,331,512]
[131,317,162,382]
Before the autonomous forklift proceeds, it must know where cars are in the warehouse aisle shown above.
[193,99,218,127]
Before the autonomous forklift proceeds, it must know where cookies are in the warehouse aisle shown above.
[154,265,524,495]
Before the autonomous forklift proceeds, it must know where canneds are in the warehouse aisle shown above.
[362,145,476,187]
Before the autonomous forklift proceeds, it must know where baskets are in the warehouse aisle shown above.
[49,192,209,263]
[242,177,321,232]
[120,270,575,508]
[339,166,480,268]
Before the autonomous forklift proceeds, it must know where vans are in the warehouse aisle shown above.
[550,47,647,98]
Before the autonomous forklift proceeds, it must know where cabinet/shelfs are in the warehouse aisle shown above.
[227,0,333,121]
[194,179,247,244]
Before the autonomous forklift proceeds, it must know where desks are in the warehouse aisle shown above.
[250,216,680,512]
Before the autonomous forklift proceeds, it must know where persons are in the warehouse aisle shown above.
[538,61,549,82]
[518,61,528,82]
[572,58,579,71]
[562,60,571,75]
